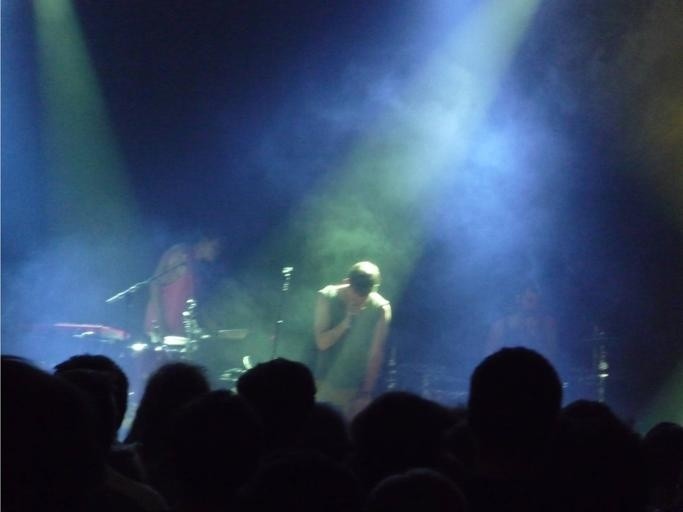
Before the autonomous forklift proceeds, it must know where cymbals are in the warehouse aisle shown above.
[55,323,130,340]
[217,329,275,340]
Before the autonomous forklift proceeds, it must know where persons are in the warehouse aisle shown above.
[1,344,683,512]
[485,275,558,364]
[296,259,392,421]
[142,242,220,341]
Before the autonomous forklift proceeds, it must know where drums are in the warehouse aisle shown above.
[577,330,635,390]
[130,336,196,406]
